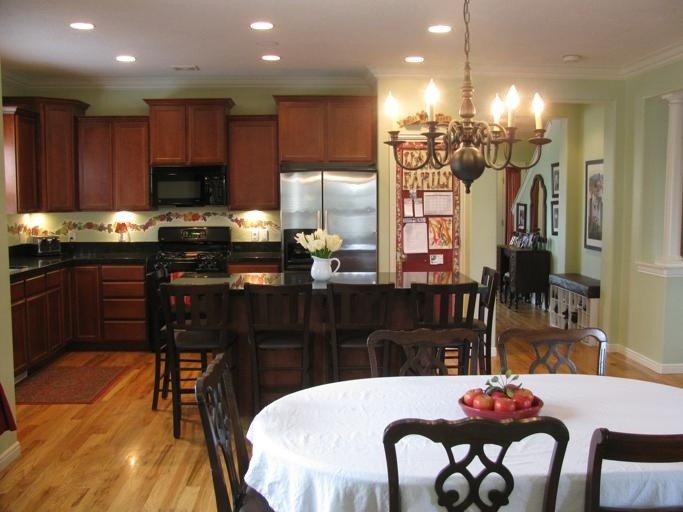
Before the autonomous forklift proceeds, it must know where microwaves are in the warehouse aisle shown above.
[149,164,228,208]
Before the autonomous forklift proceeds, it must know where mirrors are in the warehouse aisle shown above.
[528,174,548,242]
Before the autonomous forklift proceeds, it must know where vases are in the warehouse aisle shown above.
[307,257,343,283]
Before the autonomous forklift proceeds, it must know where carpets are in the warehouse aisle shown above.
[15,363,131,404]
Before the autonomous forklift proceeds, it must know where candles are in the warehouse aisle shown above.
[384,90,399,131]
[492,93,504,131]
[424,78,440,123]
[505,85,519,127]
[530,92,547,129]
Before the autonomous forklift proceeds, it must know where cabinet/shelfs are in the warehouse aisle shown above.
[75,115,148,212]
[229,263,280,274]
[272,95,378,166]
[548,272,601,349]
[142,98,235,166]
[2,96,89,214]
[10,265,67,382]
[4,107,38,214]
[72,266,148,344]
[230,114,281,210]
[496,244,551,312]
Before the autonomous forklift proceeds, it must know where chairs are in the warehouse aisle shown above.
[141,263,219,413]
[193,350,252,511]
[315,282,397,383]
[405,280,482,373]
[435,264,503,374]
[238,280,312,414]
[158,281,233,439]
[577,420,683,511]
[380,413,570,512]
[365,325,483,376]
[495,326,614,374]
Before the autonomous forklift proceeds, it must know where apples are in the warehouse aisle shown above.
[473,395,492,409]
[494,398,514,412]
[505,382,522,398]
[491,388,507,402]
[463,388,482,406]
[486,386,503,396]
[514,390,534,406]
[491,391,507,400]
[512,397,531,410]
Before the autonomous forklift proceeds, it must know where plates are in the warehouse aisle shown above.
[457,396,544,421]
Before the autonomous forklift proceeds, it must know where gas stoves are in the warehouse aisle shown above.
[145,242,231,273]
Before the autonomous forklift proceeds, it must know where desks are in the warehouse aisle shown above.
[173,271,481,373]
[251,374,679,512]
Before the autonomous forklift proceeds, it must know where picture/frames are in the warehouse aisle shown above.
[583,157,609,252]
[551,201,560,236]
[551,160,560,199]
[428,217,454,250]
[516,199,528,235]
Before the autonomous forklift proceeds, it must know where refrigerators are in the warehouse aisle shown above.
[279,163,378,285]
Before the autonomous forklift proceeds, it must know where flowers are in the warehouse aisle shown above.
[292,226,346,258]
